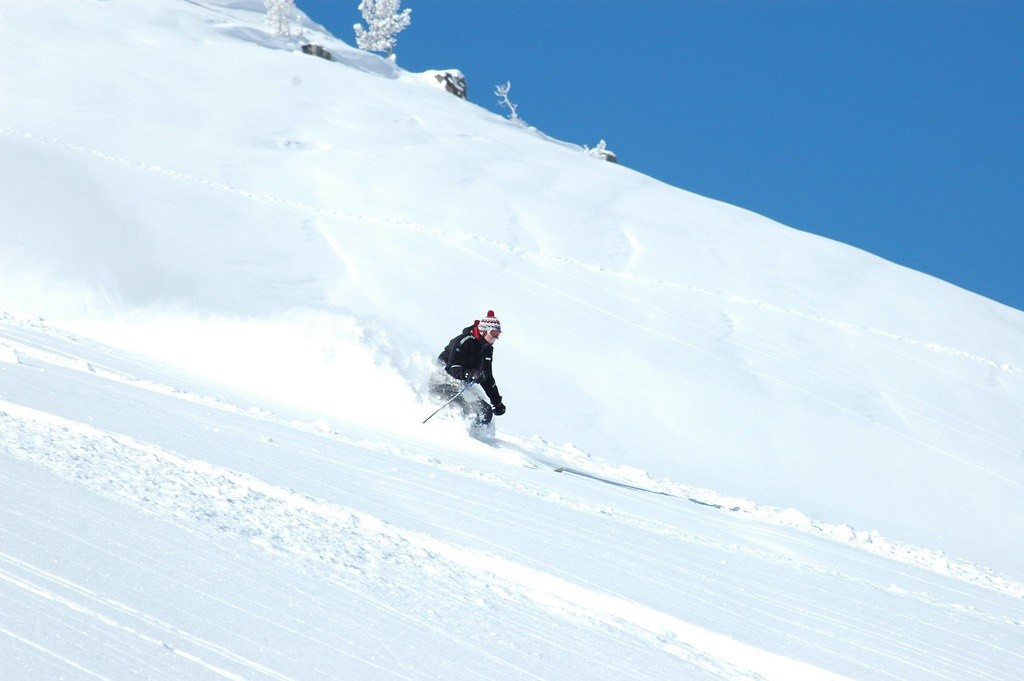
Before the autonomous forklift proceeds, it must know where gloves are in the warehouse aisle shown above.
[471,369,488,383]
[492,402,506,415]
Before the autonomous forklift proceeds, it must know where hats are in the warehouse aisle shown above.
[478,310,500,332]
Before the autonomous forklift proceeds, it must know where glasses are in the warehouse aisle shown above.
[487,329,500,339]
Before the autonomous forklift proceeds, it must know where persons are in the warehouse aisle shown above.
[429,309,506,427]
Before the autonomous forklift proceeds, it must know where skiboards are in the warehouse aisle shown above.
[524,464,565,473]
